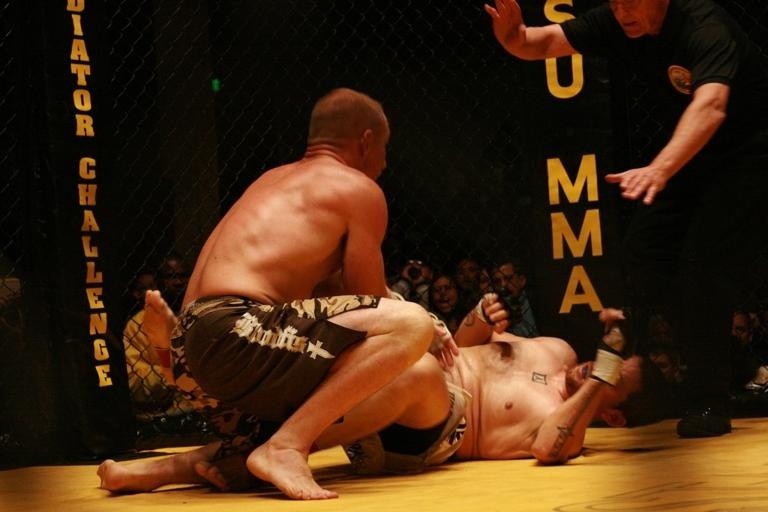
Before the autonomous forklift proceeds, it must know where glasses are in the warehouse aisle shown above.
[406,259,424,264]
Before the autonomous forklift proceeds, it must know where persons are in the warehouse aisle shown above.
[484,0,749,433]
[179,84,462,502]
[126,257,768,445]
[97,287,670,496]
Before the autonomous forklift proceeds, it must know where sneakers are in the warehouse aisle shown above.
[675,401,734,438]
